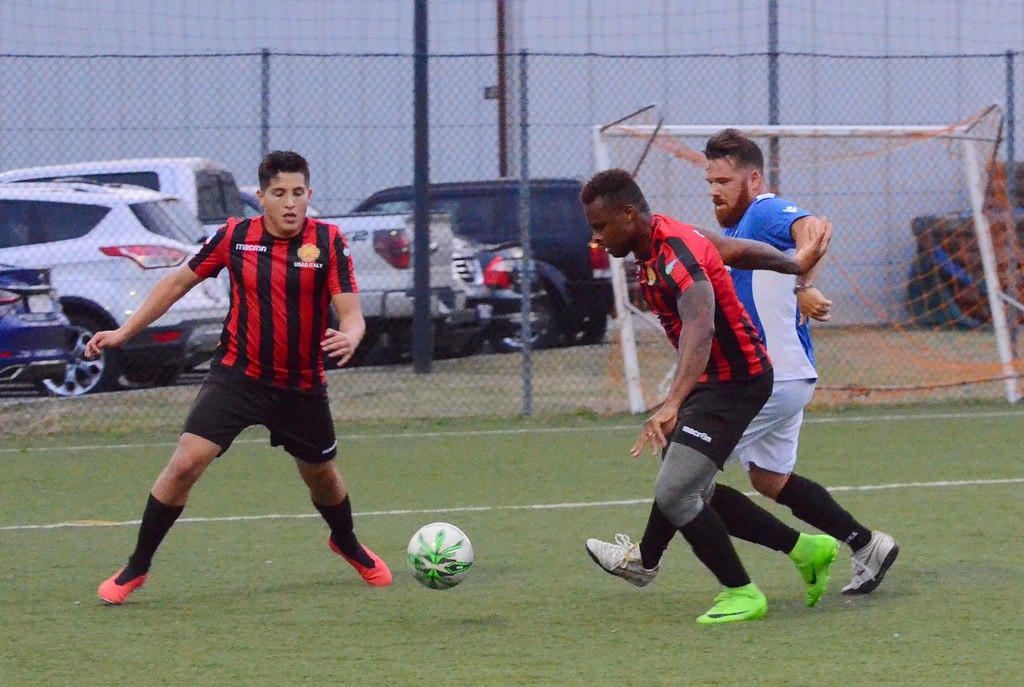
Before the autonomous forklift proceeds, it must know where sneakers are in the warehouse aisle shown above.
[329,539,393,587]
[840,529,900,595]
[98,558,148,605]
[696,584,768,625]
[586,533,659,588]
[787,532,839,610]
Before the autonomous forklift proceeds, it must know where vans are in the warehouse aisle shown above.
[353,178,652,351]
[0,157,243,295]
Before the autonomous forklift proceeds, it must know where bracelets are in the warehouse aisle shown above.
[794,284,813,293]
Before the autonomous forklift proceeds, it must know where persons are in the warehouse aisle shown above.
[584,128,900,595]
[84,150,393,603]
[581,169,840,623]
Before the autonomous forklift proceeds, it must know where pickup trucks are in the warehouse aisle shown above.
[236,184,467,367]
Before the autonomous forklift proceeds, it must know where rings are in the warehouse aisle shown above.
[646,432,655,436]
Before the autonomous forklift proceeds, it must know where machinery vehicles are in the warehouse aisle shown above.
[0,175,231,399]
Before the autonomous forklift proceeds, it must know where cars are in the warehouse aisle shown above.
[459,236,548,356]
[0,263,76,386]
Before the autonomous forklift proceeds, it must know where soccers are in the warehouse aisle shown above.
[406,521,474,591]
